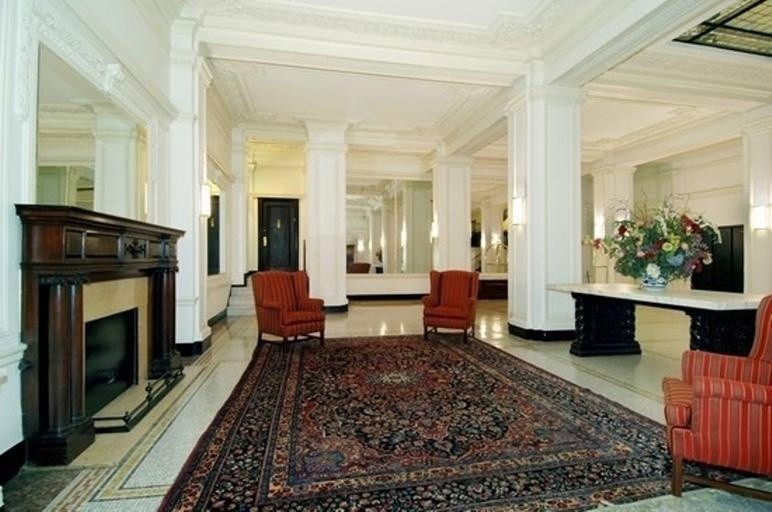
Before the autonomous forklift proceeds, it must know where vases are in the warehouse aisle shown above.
[639,270,669,291]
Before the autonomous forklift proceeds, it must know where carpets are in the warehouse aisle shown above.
[154,331,757,511]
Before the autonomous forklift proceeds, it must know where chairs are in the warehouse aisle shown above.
[251,271,326,354]
[660,293,771,503]
[421,270,479,343]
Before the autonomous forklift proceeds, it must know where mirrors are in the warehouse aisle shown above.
[472,178,507,274]
[344,176,434,275]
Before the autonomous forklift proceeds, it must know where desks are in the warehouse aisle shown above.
[544,282,762,360]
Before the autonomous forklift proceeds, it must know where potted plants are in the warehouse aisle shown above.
[581,186,723,279]
